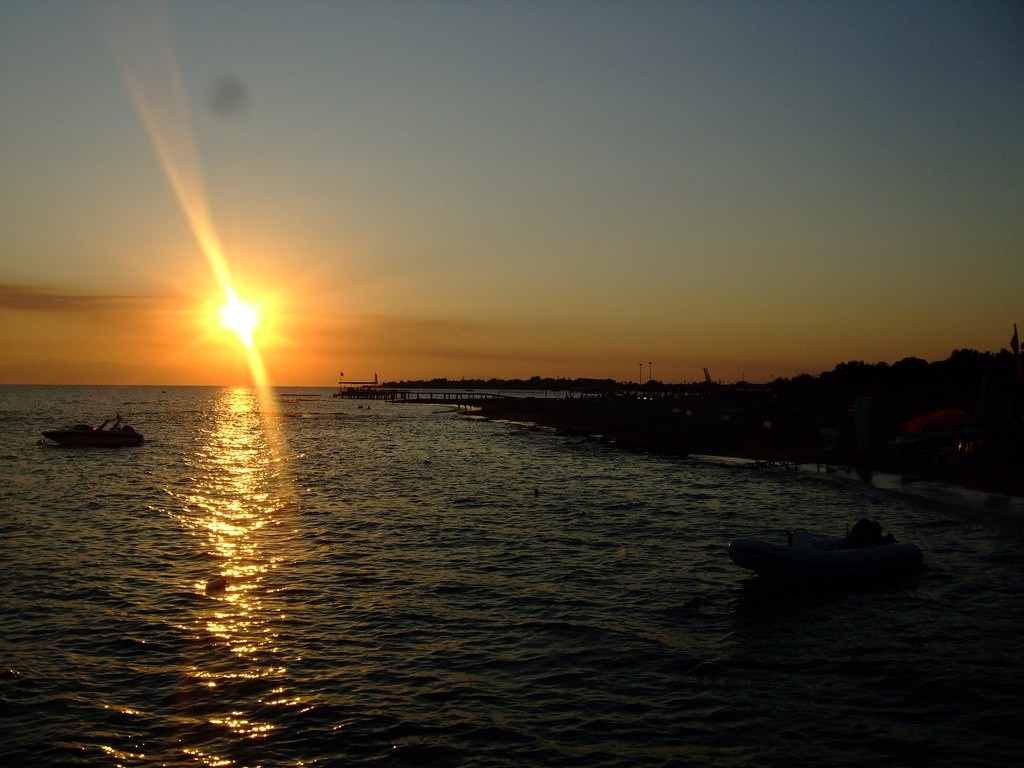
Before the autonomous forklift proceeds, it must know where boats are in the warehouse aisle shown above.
[41,414,145,448]
[725,518,921,581]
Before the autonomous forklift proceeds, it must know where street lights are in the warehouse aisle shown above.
[639,364,642,385]
[649,362,652,380]
